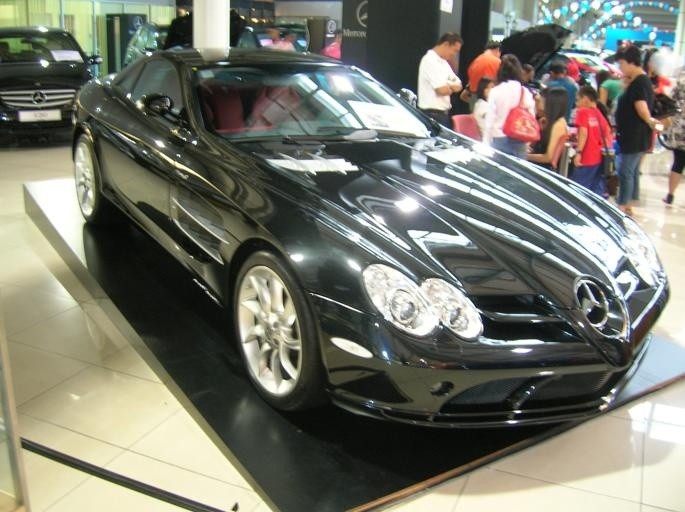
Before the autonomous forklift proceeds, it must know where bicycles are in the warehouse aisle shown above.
[656,89,685,152]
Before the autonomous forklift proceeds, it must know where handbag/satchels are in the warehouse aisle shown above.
[603,159,614,177]
[503,85,541,142]
[656,113,685,151]
[651,93,677,120]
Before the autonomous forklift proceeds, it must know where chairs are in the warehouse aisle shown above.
[200,77,319,136]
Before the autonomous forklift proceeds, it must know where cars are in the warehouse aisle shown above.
[1,26,102,143]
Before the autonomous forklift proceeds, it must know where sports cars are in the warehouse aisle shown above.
[69,49,674,433]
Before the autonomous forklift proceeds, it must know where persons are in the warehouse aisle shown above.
[416,30,463,129]
[571,86,608,197]
[638,47,672,176]
[527,86,569,172]
[271,31,297,52]
[612,46,672,214]
[486,53,534,161]
[663,71,685,204]
[321,29,342,61]
[466,40,501,115]
[473,76,495,140]
[513,60,627,121]
